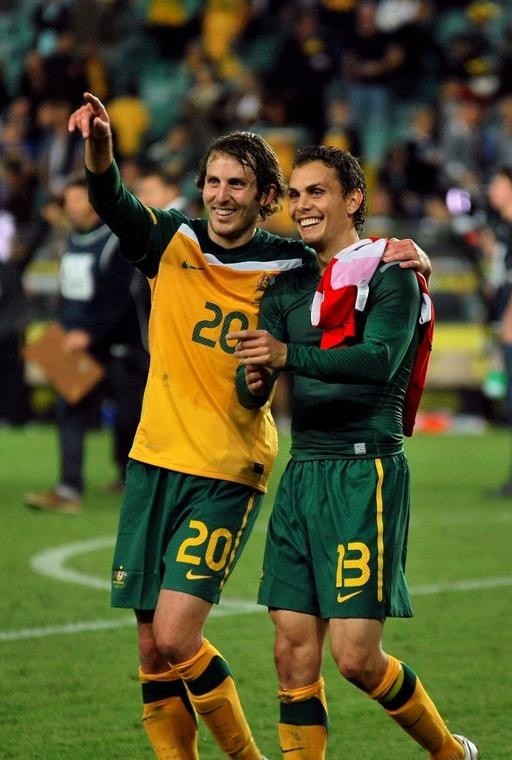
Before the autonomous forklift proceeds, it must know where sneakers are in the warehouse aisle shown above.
[23,489,81,516]
[451,733,480,760]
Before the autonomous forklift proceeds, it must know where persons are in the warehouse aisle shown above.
[226,140,485,760]
[67,90,433,759]
[18,170,147,515]
[1,1,512,443]
